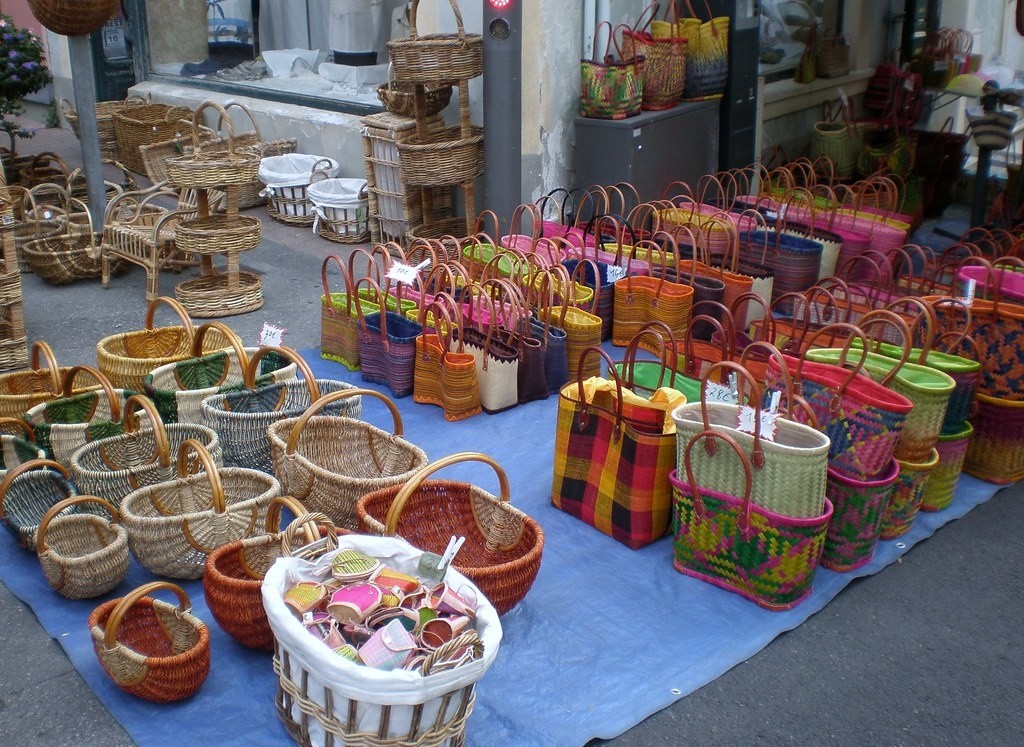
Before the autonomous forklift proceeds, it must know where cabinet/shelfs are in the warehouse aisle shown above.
[575,98,724,223]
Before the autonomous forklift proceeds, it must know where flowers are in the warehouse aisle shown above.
[0,14,53,151]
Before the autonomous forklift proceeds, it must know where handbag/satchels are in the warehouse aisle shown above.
[320,2,1024,611]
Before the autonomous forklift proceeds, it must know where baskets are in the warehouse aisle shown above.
[0,0,544,747]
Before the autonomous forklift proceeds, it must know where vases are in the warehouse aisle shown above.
[4,156,51,185]
